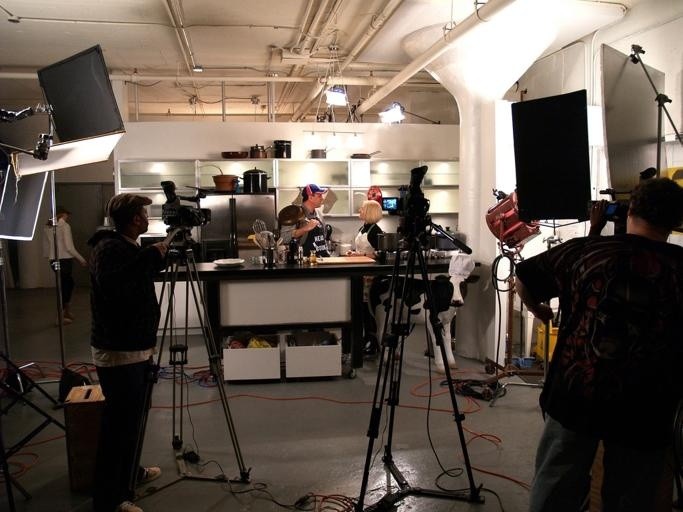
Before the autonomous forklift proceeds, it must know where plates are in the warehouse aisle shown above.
[213,259,245,267]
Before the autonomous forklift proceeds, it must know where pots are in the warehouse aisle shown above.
[239,167,273,193]
[273,141,290,158]
[275,204,319,227]
[307,145,329,159]
[211,175,239,191]
[377,232,400,250]
[434,227,458,250]
[249,144,273,157]
[351,151,380,158]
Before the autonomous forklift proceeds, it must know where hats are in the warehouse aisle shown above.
[108,193,153,218]
[302,183,328,195]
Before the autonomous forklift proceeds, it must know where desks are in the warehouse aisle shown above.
[155,257,480,384]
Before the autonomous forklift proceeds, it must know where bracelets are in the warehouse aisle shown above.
[364,251,366,257]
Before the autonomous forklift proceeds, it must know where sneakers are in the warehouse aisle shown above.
[118,500,143,512]
[138,466,162,485]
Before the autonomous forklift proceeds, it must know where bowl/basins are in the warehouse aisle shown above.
[221,152,248,159]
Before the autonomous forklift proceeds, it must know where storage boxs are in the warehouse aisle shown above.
[223,335,281,381]
[63,384,105,489]
[285,328,342,378]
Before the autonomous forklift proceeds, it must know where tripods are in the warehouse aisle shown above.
[126,248,252,503]
[355,238,485,512]
[485,243,544,385]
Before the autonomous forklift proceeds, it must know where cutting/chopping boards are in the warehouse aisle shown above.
[314,255,376,264]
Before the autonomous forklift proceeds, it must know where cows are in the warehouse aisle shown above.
[368,274,480,376]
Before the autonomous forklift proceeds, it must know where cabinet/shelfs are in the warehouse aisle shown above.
[116,158,459,248]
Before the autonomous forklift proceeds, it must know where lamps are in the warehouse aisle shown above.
[326,86,349,108]
[378,101,441,124]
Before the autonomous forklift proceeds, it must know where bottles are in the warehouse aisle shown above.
[289,237,298,263]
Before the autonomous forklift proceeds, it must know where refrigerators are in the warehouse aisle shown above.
[198,192,279,256]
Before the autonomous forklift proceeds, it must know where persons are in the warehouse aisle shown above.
[89,193,169,511]
[42,205,85,324]
[346,199,384,358]
[278,184,329,254]
[515,178,683,511]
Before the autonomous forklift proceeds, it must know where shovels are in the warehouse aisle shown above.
[253,219,266,234]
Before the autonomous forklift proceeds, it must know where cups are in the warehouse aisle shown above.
[260,248,277,264]
[308,250,316,265]
[340,245,351,255]
